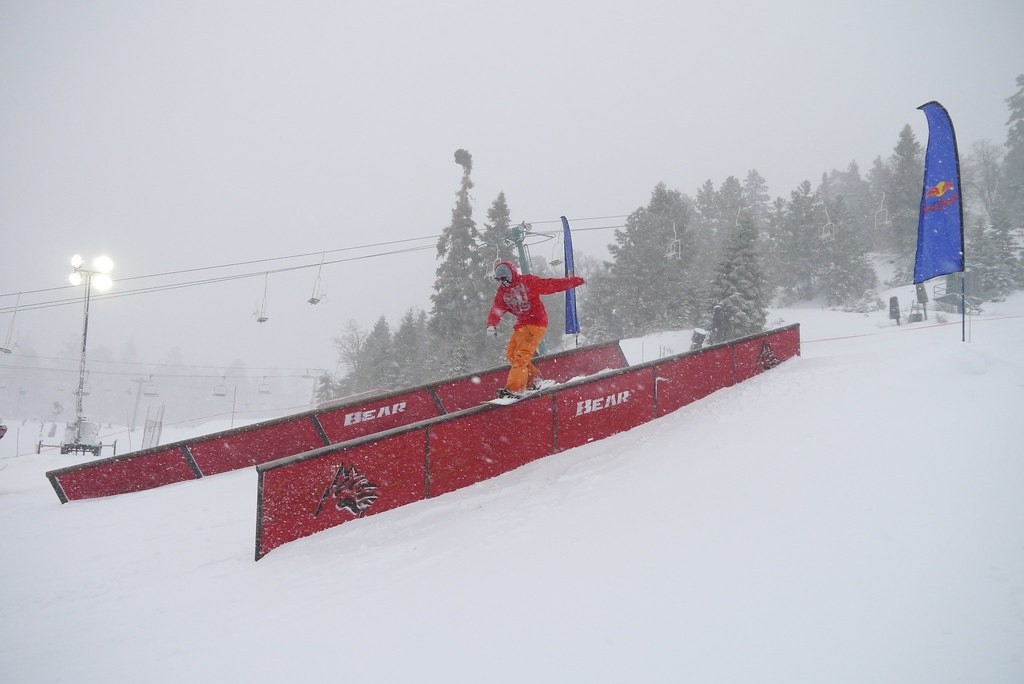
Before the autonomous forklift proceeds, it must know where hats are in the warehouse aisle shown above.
[495,264,511,279]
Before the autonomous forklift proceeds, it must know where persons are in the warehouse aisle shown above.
[486,262,585,397]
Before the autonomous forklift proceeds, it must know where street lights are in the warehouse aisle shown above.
[59,253,115,457]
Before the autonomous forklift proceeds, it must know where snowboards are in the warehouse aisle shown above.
[478,378,555,406]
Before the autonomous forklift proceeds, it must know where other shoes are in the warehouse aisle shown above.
[533,377,543,390]
[497,389,520,399]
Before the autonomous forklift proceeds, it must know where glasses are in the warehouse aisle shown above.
[495,277,507,280]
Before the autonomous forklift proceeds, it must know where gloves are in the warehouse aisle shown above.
[486,325,498,336]
